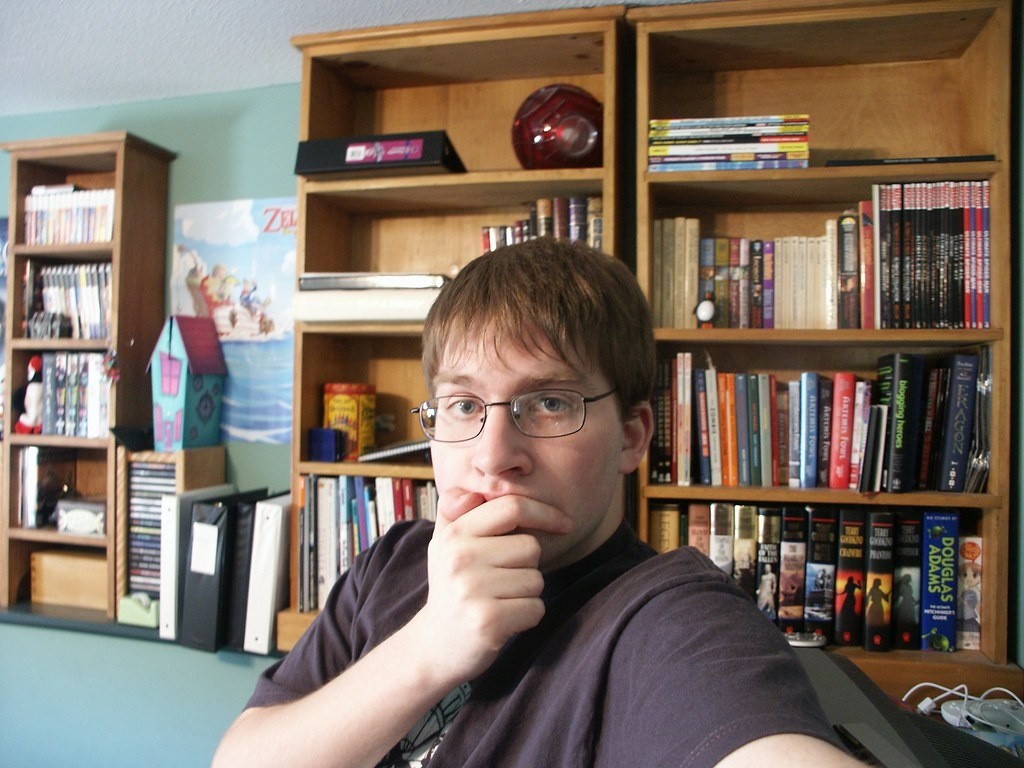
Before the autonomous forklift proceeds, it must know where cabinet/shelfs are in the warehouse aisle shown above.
[625,1,1012,666]
[116,444,224,628]
[276,3,637,650]
[1,130,177,625]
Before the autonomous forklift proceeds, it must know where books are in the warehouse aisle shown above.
[648,344,992,494]
[356,437,432,466]
[38,262,112,340]
[480,196,603,290]
[649,501,982,655]
[295,272,453,323]
[647,114,995,171]
[23,181,115,246]
[14,443,79,535]
[295,466,442,615]
[649,180,991,329]
[40,351,112,438]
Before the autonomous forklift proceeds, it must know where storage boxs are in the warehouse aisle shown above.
[158,482,292,657]
[30,549,108,613]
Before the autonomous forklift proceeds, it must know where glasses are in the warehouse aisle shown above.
[409,386,621,443]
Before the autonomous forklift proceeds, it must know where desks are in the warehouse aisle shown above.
[847,660,1023,768]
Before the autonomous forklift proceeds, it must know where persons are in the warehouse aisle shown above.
[203,237,869,768]
